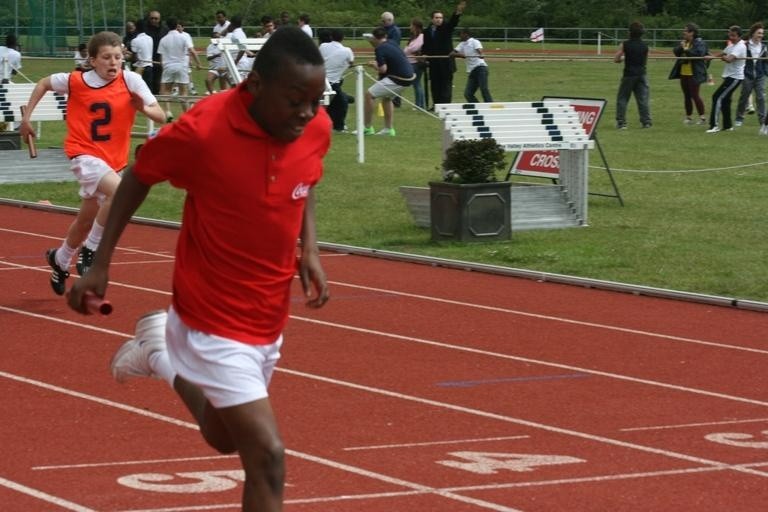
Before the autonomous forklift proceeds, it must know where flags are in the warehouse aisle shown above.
[529,27,544,42]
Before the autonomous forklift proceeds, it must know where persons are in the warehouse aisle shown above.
[0,33,22,84]
[67,23,333,511]
[614,21,653,130]
[74,10,356,136]
[351,4,493,138]
[668,23,768,135]
[20,30,171,296]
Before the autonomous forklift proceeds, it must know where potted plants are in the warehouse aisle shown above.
[430,137,513,243]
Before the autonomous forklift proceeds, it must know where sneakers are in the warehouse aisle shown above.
[377,128,395,136]
[77,246,95,275]
[351,128,375,134]
[44,248,68,294]
[110,310,167,383]
[706,126,719,133]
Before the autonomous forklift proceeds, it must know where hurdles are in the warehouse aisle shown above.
[0,83,82,183]
[400,100,596,231]
[209,36,337,106]
